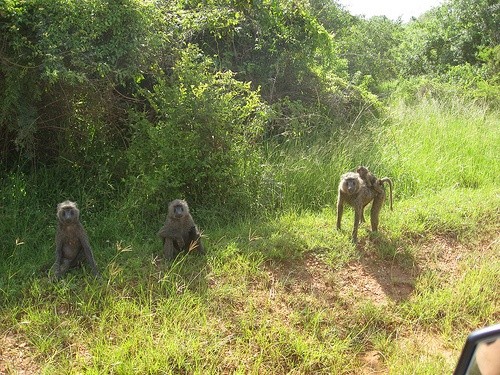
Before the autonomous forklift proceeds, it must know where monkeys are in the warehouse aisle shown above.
[50,199,100,283]
[158,197,207,259]
[336,166,393,244]
